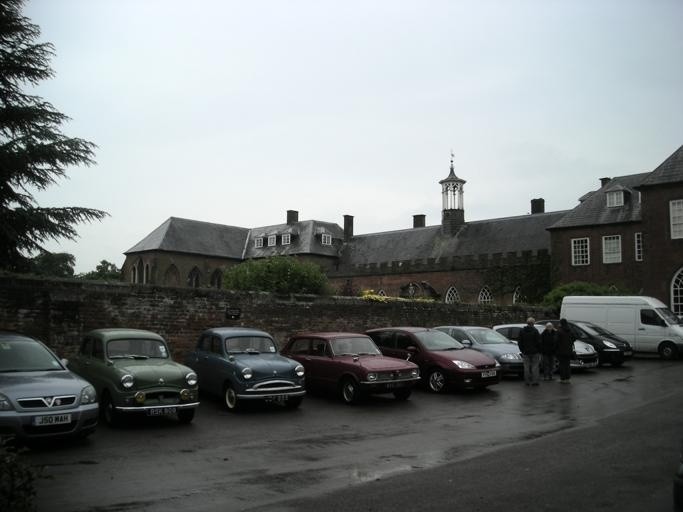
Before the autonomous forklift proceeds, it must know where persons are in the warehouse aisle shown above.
[555,319,575,385]
[517,317,540,387]
[540,322,557,381]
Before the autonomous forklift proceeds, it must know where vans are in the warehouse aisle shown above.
[559,295,683,360]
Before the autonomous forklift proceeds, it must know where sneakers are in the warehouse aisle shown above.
[524,376,572,386]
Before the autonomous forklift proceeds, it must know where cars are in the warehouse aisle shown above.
[0,331,100,447]
[362,326,502,392]
[535,319,632,368]
[190,327,310,410]
[432,326,524,380]
[66,326,199,425]
[492,323,600,369]
[278,331,421,403]
